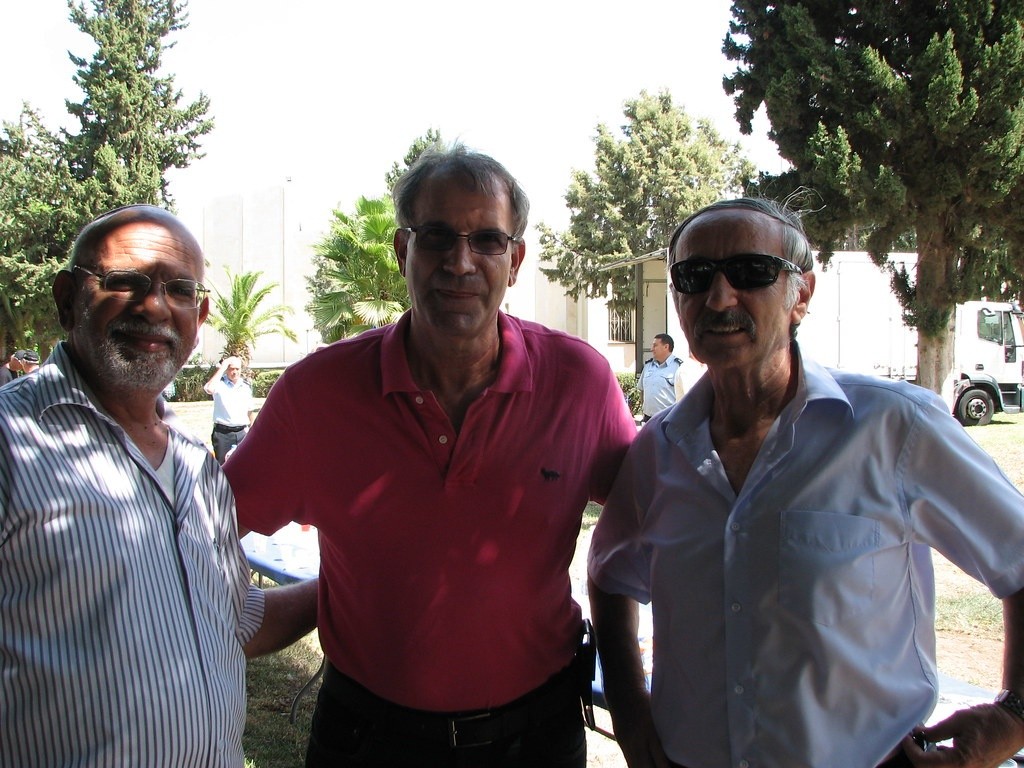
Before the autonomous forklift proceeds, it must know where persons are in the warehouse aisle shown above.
[1,205,319,768]
[203,356,255,467]
[636,333,712,425]
[586,195,1023,768]
[0,348,41,387]
[223,146,639,768]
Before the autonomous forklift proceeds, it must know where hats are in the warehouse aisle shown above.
[23,352,40,363]
[15,349,26,361]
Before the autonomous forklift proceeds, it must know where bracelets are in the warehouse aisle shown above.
[994,688,1023,718]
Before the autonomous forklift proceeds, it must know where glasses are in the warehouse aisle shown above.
[71,265,212,310]
[669,253,804,296]
[402,224,517,255]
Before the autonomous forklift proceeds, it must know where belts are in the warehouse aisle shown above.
[322,662,583,749]
[214,423,244,432]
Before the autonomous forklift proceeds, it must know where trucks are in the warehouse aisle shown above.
[667,248,1024,427]
